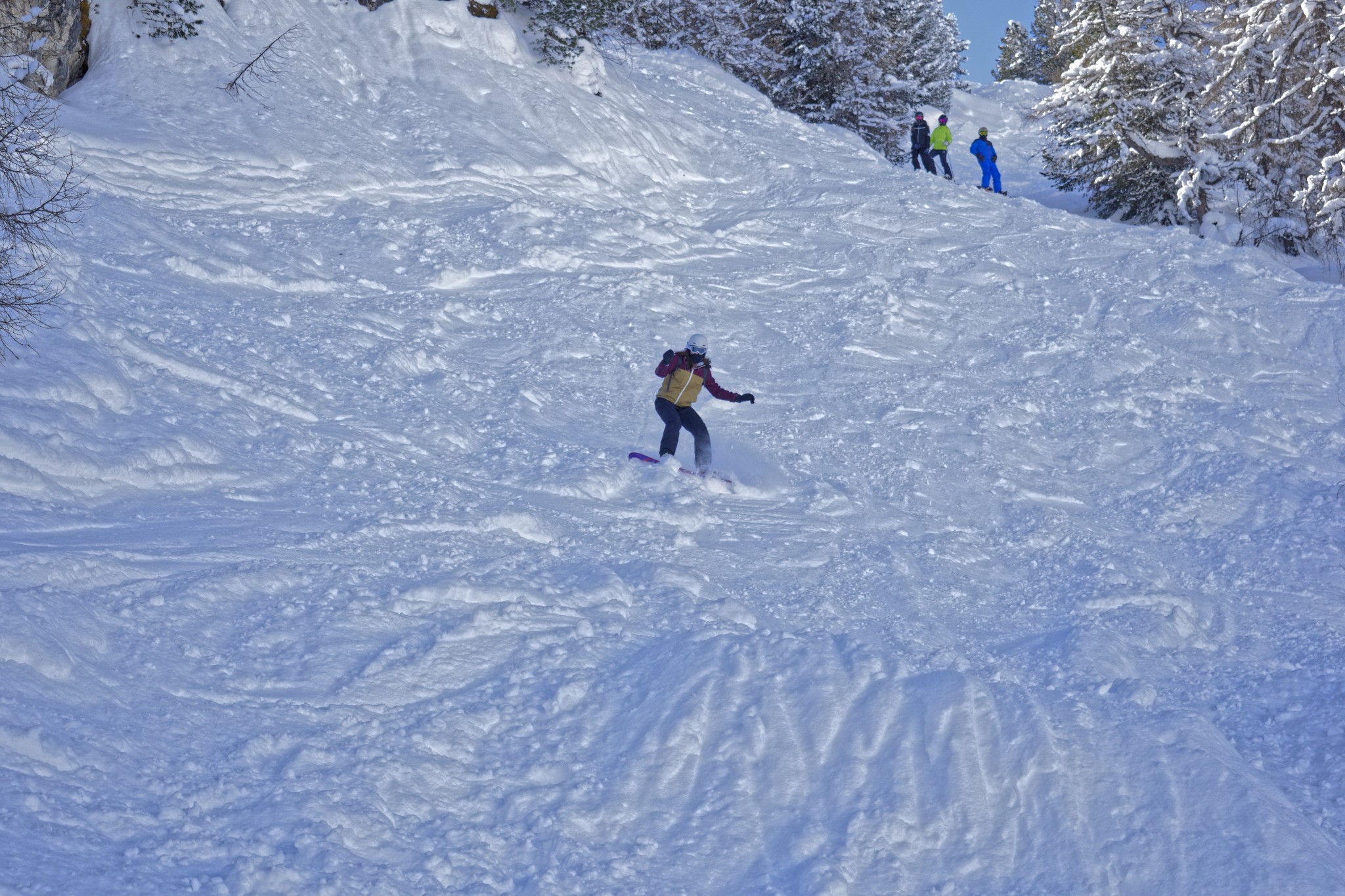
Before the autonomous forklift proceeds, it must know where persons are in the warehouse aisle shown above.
[910,112,934,174]
[654,334,756,475]
[930,114,953,179]
[969,128,1008,195]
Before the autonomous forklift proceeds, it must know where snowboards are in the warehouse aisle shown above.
[627,451,733,490]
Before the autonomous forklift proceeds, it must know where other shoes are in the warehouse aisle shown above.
[999,191,1006,195]
[945,173,952,178]
[985,186,993,191]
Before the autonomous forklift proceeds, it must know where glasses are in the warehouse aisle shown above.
[980,131,987,136]
[689,345,706,355]
[939,118,946,123]
[915,116,923,120]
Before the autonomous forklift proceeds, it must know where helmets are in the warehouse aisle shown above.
[687,334,708,353]
[939,115,947,126]
[978,127,988,134]
[915,112,923,123]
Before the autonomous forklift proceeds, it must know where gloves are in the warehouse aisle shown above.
[914,144,920,150]
[735,393,755,404]
[944,141,949,146]
[662,349,674,365]
[977,154,985,162]
[992,155,997,162]
[924,146,930,154]
